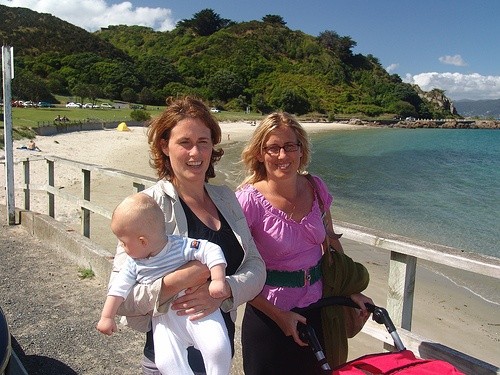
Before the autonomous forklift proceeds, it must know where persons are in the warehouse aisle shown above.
[109,97,266,375]
[29,140,41,152]
[235,111,374,375]
[96,191,232,375]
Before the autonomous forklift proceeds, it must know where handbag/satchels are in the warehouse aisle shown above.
[327,255,371,338]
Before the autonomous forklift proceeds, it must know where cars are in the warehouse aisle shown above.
[65,101,115,109]
[11,100,56,108]
[210,108,221,114]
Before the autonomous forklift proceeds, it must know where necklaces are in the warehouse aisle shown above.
[287,180,298,204]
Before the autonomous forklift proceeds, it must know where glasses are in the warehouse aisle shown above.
[261,142,302,155]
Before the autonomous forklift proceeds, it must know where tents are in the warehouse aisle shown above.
[117,122,128,131]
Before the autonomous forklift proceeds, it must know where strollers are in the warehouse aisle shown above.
[288,296,467,375]
